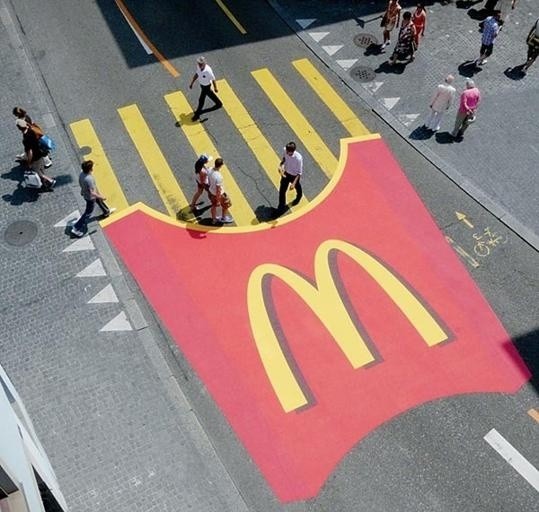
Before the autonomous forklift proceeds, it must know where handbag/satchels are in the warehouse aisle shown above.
[23,169,43,189]
[39,135,56,152]
[527,34,539,50]
[30,123,44,140]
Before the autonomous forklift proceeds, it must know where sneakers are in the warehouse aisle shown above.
[71,227,84,237]
[211,216,234,225]
[213,103,222,109]
[425,120,464,141]
[188,206,199,213]
[276,196,302,213]
[379,39,418,70]
[192,116,200,121]
[16,153,57,189]
[103,207,117,216]
[477,57,488,65]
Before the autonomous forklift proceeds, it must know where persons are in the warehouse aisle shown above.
[207,157,233,225]
[16,119,56,191]
[276,142,303,214]
[451,79,482,139]
[381,0,521,66]
[423,73,456,131]
[71,159,117,238]
[189,153,209,210]
[11,106,52,169]
[189,55,222,121]
[520,16,539,73]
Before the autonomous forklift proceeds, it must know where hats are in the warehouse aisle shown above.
[197,56,208,65]
[200,154,213,162]
